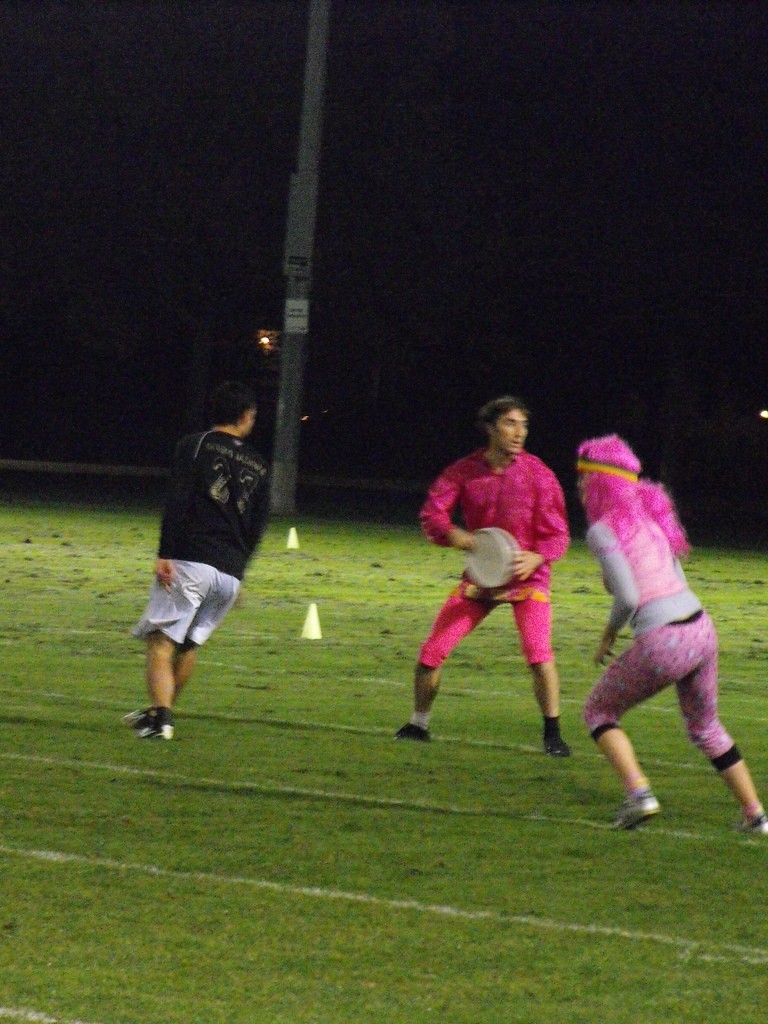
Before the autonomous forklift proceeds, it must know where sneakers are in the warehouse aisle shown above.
[393,722,431,743]
[543,737,570,757]
[736,810,768,835]
[129,706,175,740]
[610,788,662,831]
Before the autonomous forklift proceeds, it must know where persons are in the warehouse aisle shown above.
[576,433,768,837]
[397,396,572,757]
[120,379,271,740]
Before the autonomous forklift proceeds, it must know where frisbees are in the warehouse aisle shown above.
[466,528,522,589]
